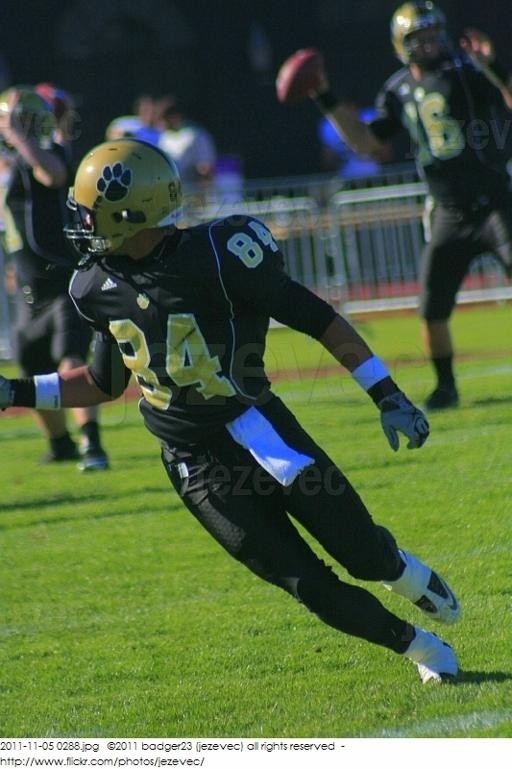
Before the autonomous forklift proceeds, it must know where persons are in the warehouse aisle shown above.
[0,85,111,473]
[0,140,462,685]
[274,1,512,409]
[1,63,512,353]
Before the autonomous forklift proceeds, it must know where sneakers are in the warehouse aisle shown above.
[41,444,109,472]
[425,388,458,409]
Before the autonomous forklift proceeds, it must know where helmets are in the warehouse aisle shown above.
[391,1,451,65]
[0,83,73,151]
[63,138,184,257]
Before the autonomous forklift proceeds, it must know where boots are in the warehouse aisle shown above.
[403,628,459,684]
[382,549,460,625]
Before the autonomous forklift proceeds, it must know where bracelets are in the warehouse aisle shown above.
[349,354,391,393]
[31,370,62,413]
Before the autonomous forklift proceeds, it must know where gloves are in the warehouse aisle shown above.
[367,376,429,451]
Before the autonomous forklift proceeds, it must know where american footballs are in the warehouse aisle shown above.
[275,48,325,105]
